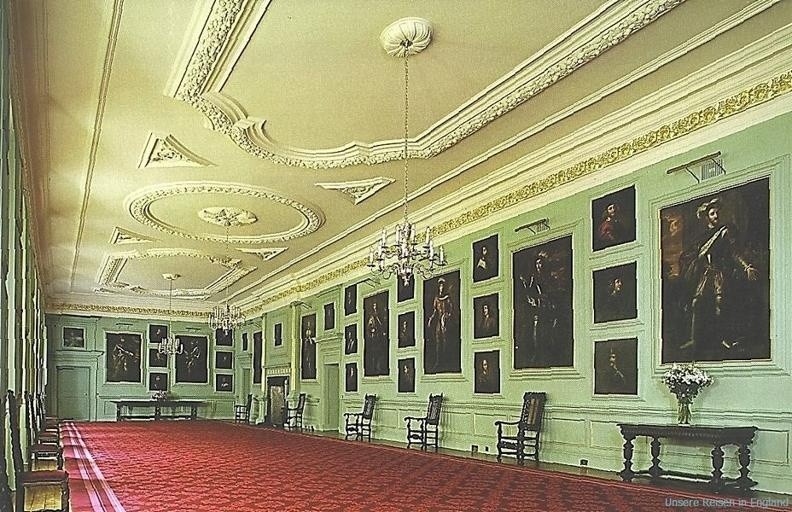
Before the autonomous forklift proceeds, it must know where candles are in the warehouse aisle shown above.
[369,246,374,263]
[411,223,416,241]
[430,239,433,258]
[424,226,430,244]
[377,240,381,256]
[381,227,386,245]
[439,245,444,261]
[395,223,400,242]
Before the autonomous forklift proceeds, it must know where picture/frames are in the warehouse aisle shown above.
[344,360,359,393]
[590,329,646,402]
[146,323,170,346]
[322,301,337,332]
[299,310,320,385]
[342,283,360,320]
[213,372,236,394]
[61,326,87,352]
[468,228,504,289]
[507,220,585,381]
[147,371,170,393]
[342,321,360,358]
[147,347,170,370]
[250,329,265,386]
[471,348,504,397]
[647,154,791,380]
[585,253,645,332]
[393,271,418,307]
[420,257,467,383]
[213,328,237,349]
[471,287,504,345]
[240,333,248,352]
[396,309,418,352]
[273,321,284,347]
[361,287,392,381]
[587,183,645,259]
[172,334,211,386]
[102,330,144,386]
[395,356,419,397]
[212,349,235,372]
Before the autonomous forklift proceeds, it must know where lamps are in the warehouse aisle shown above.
[664,150,728,182]
[197,207,258,335]
[366,15,447,280]
[157,273,184,357]
[515,216,553,233]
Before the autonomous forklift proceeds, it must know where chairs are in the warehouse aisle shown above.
[232,393,252,424]
[494,392,547,465]
[343,393,379,444]
[40,394,59,421]
[23,390,65,471]
[5,389,70,512]
[403,392,443,453]
[37,394,60,432]
[281,393,306,433]
[29,394,60,459]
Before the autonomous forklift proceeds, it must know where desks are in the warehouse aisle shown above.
[107,398,210,423]
[613,421,759,494]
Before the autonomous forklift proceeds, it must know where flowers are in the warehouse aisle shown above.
[661,361,714,424]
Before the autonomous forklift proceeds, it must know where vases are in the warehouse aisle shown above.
[678,400,693,424]
